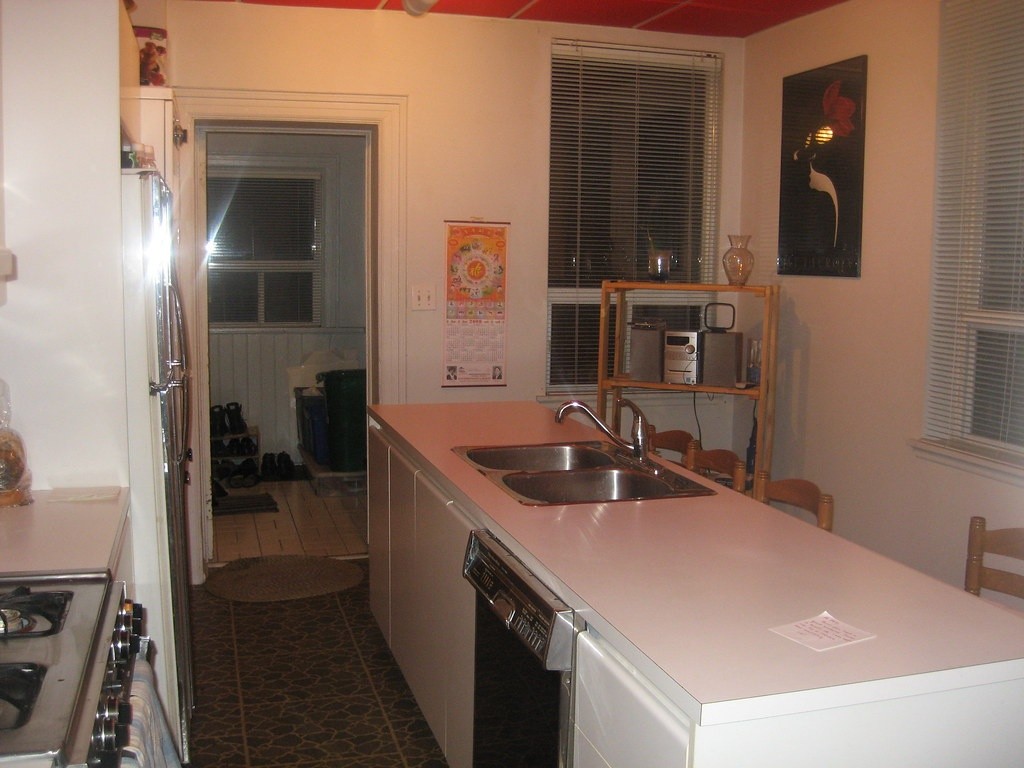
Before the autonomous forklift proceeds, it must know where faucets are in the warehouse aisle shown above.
[554,394,651,463]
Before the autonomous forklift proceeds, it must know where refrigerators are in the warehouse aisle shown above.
[121,170,195,764]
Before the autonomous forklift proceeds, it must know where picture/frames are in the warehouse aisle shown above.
[775,54,869,276]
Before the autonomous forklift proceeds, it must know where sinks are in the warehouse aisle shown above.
[464,443,619,471]
[501,470,678,504]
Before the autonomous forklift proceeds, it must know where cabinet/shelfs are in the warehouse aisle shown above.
[363,424,473,768]
[596,278,779,497]
[574,624,1024,768]
[213,426,262,480]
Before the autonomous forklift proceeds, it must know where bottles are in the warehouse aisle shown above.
[122,141,154,169]
[723,236,753,285]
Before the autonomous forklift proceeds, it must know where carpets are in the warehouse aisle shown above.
[204,554,363,604]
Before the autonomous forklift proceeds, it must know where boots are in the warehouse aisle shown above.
[211,405,228,438]
[226,402,248,433]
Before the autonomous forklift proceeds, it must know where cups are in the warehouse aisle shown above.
[648,252,669,284]
[746,339,762,382]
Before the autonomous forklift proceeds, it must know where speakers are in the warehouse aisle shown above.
[701,331,743,388]
[629,327,666,383]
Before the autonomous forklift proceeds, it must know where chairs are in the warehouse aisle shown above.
[757,477,834,528]
[641,421,694,460]
[959,517,1024,608]
[685,440,748,491]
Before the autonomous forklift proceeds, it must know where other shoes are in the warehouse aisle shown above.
[210,436,295,497]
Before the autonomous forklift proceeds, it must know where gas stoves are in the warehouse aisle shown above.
[0,571,144,768]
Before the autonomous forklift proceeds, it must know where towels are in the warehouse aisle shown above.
[120,658,185,768]
